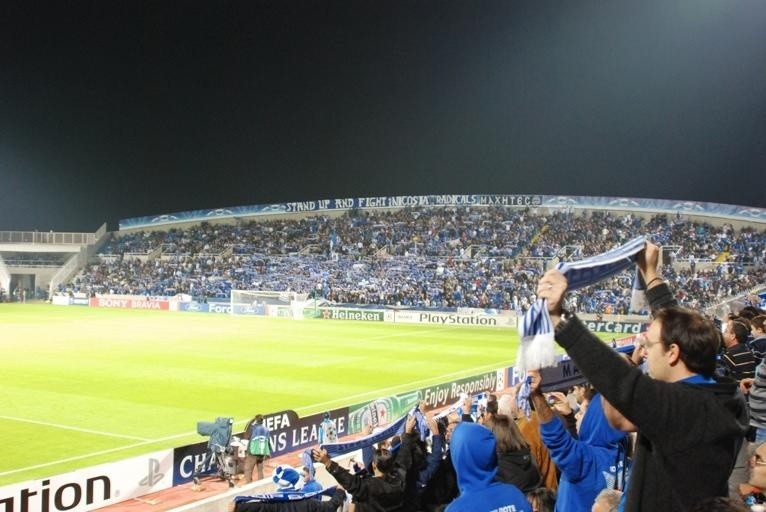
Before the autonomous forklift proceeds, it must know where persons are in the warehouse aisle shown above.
[195,240,765,512]
[1,202,764,320]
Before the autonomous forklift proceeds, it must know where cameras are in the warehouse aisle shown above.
[547,395,557,405]
